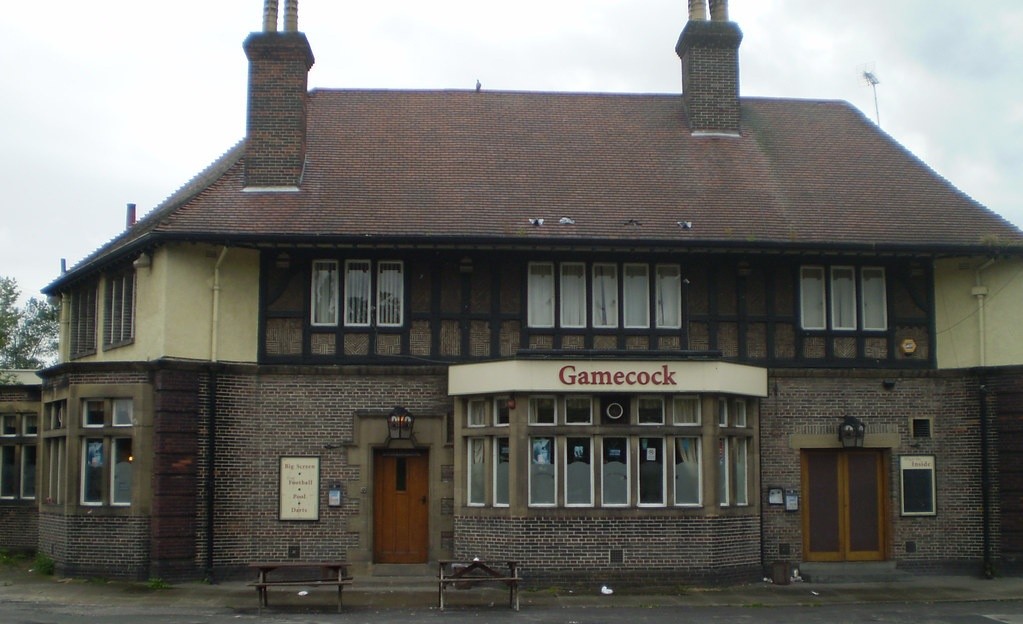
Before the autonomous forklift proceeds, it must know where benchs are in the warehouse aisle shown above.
[438,575,523,582]
[247,578,354,585]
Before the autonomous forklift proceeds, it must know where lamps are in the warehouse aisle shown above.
[382,406,419,449]
[132,252,151,268]
[838,414,866,450]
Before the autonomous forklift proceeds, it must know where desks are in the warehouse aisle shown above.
[437,557,524,612]
[245,561,354,614]
